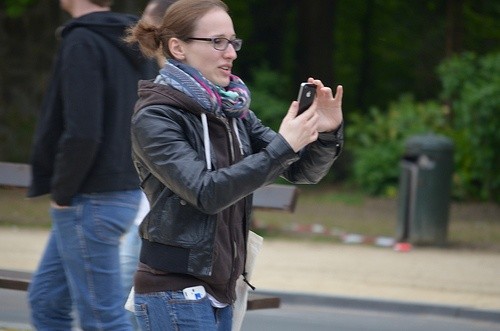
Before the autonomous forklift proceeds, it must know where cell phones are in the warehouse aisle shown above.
[182,284,207,301]
[296,81,317,117]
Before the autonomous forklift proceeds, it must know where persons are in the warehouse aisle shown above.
[25,0,151,330]
[125,0,345,331]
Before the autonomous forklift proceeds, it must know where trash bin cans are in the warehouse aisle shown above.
[395,135,455,247]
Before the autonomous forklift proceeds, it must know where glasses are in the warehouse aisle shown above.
[187,37,243,51]
